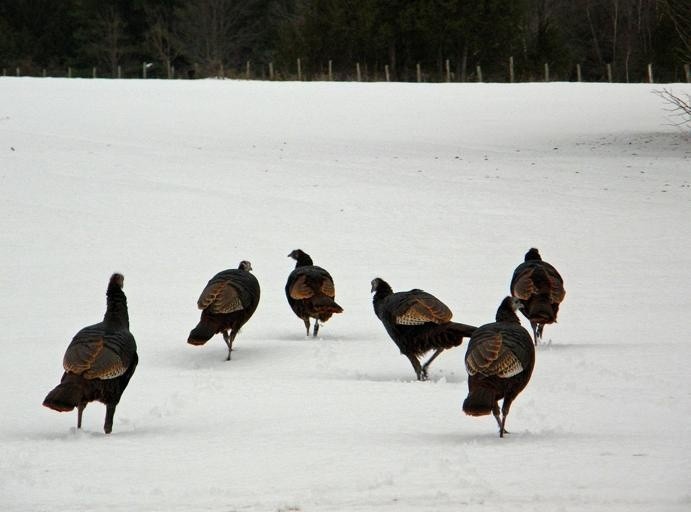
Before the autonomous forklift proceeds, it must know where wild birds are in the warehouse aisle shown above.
[463,296,535,438]
[188,261,260,360]
[511,248,566,346]
[43,274,138,434]
[285,249,344,336]
[371,278,478,380]
